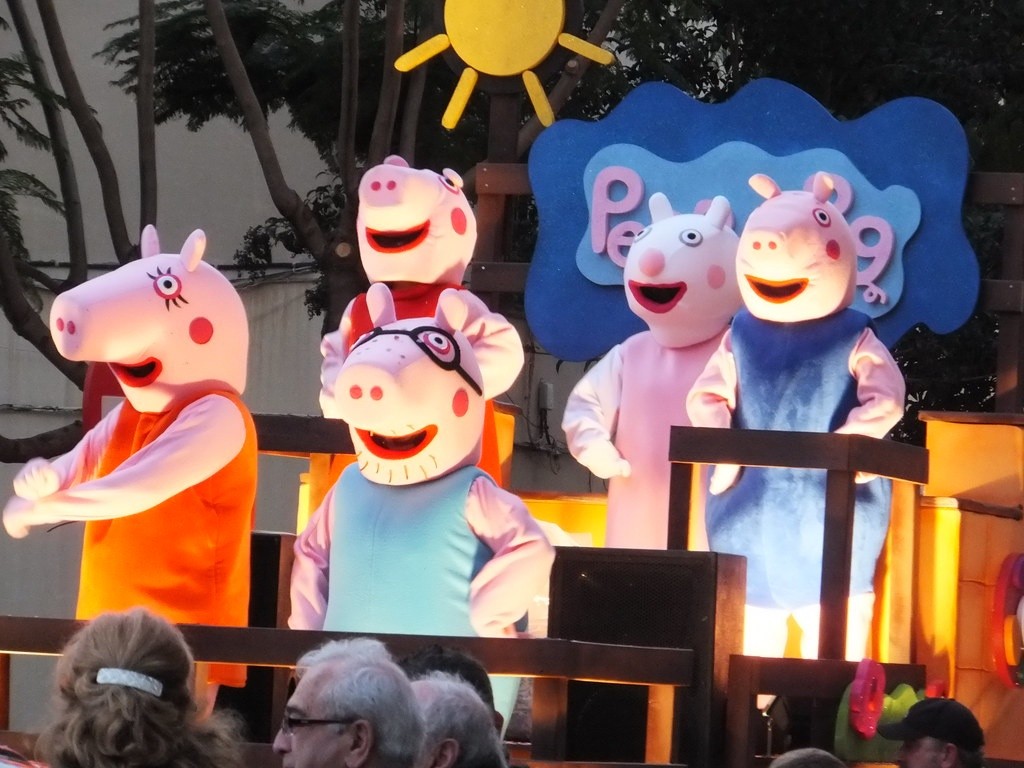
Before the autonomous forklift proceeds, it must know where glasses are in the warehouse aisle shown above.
[280,715,353,736]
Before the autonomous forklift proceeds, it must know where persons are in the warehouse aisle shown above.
[410,672,508,768]
[272,637,427,768]
[34,609,242,767]
[397,646,504,740]
[772,697,985,768]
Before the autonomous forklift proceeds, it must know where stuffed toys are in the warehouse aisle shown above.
[562,172,906,709]
[4,225,257,720]
[289,153,554,740]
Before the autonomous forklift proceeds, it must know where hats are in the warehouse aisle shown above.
[396,645,494,713]
[877,697,983,745]
